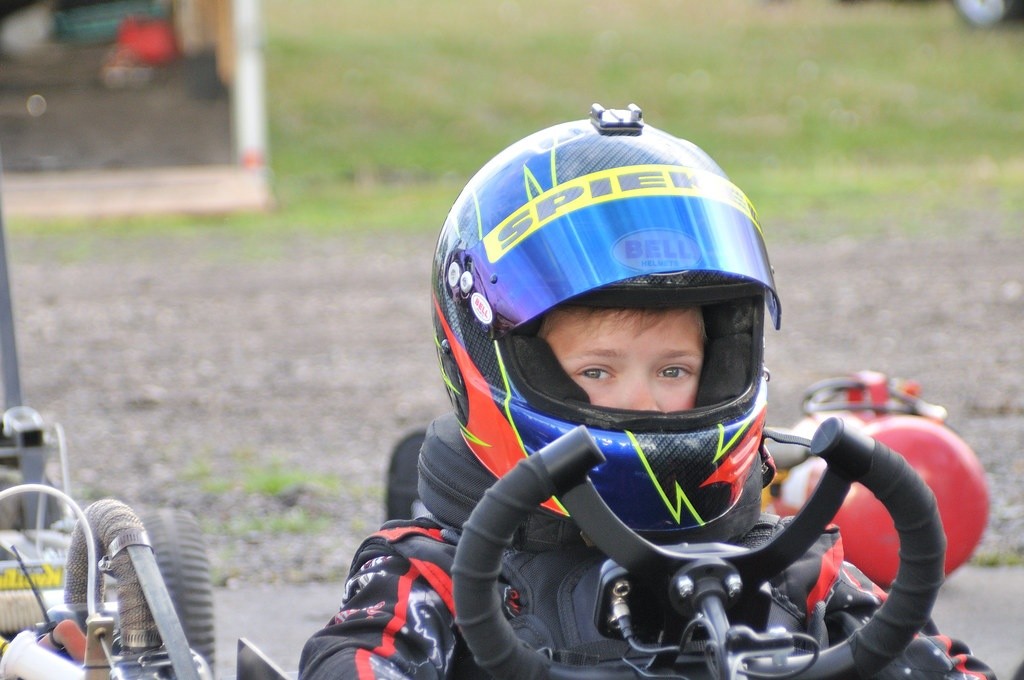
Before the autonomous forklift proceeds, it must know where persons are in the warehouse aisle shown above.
[300,114,995,680]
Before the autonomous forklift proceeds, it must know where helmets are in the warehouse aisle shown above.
[432,103,782,548]
